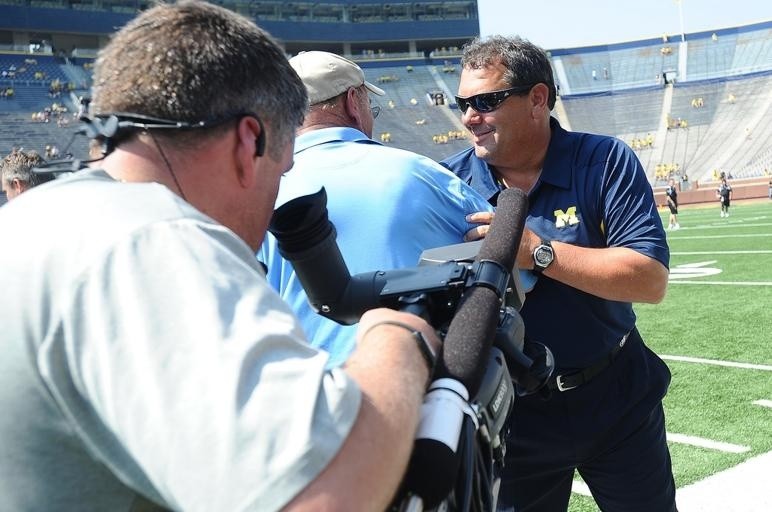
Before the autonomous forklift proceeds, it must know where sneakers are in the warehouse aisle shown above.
[668,224,674,231]
[718,211,731,218]
[675,223,681,230]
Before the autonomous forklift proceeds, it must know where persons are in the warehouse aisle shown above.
[655,72,660,83]
[691,97,705,112]
[603,68,610,78]
[653,159,687,235]
[660,44,673,56]
[1,150,55,202]
[664,113,691,133]
[0,2,442,512]
[590,69,599,80]
[437,32,677,511]
[253,51,538,379]
[745,128,751,140]
[624,132,653,151]
[712,168,732,218]
[1,58,91,162]
[763,168,772,200]
[358,42,469,146]
[712,32,718,43]
[727,90,735,106]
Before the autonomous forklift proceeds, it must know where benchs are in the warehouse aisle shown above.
[1,23,772,189]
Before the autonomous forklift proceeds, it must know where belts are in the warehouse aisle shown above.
[500,324,641,395]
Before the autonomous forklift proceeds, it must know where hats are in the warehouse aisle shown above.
[268,48,386,104]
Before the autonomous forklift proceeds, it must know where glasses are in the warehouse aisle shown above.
[453,81,553,110]
[345,99,384,120]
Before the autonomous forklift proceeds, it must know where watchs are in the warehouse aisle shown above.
[531,239,556,277]
[360,321,441,392]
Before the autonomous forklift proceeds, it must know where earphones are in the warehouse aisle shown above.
[254,131,267,158]
